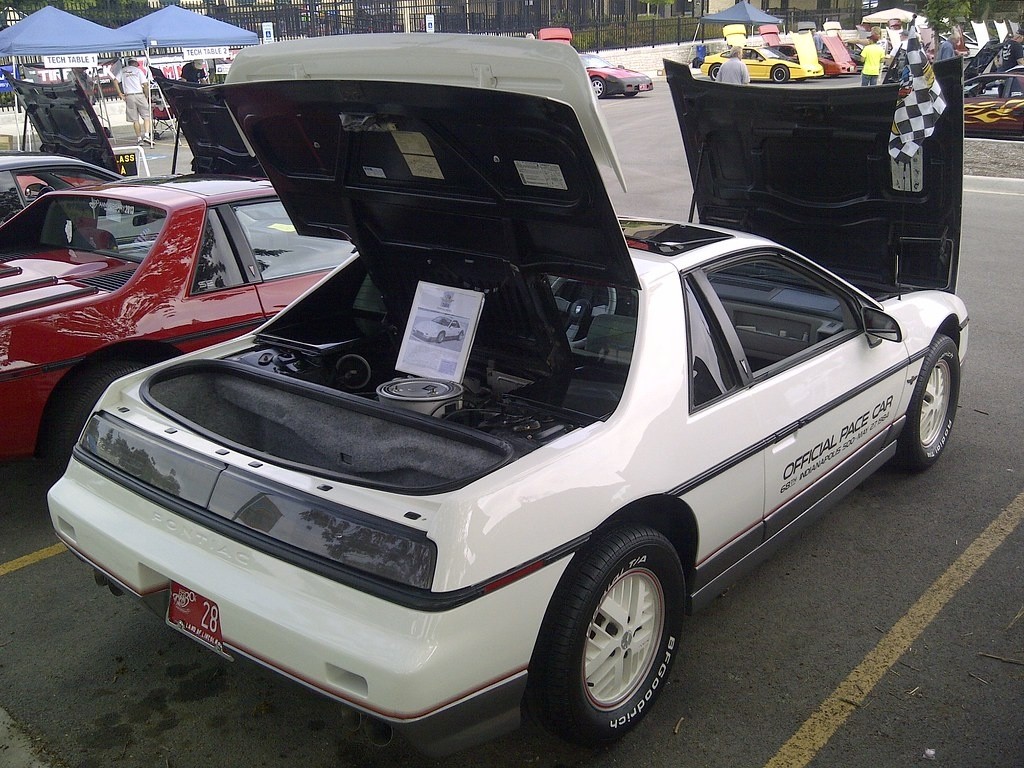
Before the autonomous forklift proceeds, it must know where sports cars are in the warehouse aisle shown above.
[1,168,361,484]
[760,25,858,78]
[576,51,655,99]
[44,29,971,750]
[701,24,824,84]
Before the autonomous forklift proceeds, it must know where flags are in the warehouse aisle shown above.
[888,25,948,165]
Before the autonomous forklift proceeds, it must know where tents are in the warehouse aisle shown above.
[860,8,929,29]
[0,4,260,151]
[687,0,786,61]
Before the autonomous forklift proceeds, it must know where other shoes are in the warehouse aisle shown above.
[143,137,156,145]
[136,139,144,146]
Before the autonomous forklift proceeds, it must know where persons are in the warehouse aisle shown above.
[112,57,156,146]
[180,59,207,84]
[859,32,885,86]
[715,46,750,85]
[995,29,1024,98]
[889,31,909,66]
[936,31,960,60]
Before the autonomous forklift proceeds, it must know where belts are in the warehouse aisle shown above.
[123,93,144,97]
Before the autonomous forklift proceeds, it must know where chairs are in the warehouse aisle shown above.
[153,106,179,139]
[73,227,119,254]
[584,313,637,362]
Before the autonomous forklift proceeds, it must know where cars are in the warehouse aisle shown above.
[964,72,1024,142]
[0,148,128,250]
[963,65,1024,97]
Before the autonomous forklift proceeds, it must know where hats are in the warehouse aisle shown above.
[194,59,204,69]
[1016,29,1024,35]
[867,35,878,41]
[890,22,900,27]
[938,30,952,41]
[899,30,908,36]
[128,57,140,67]
